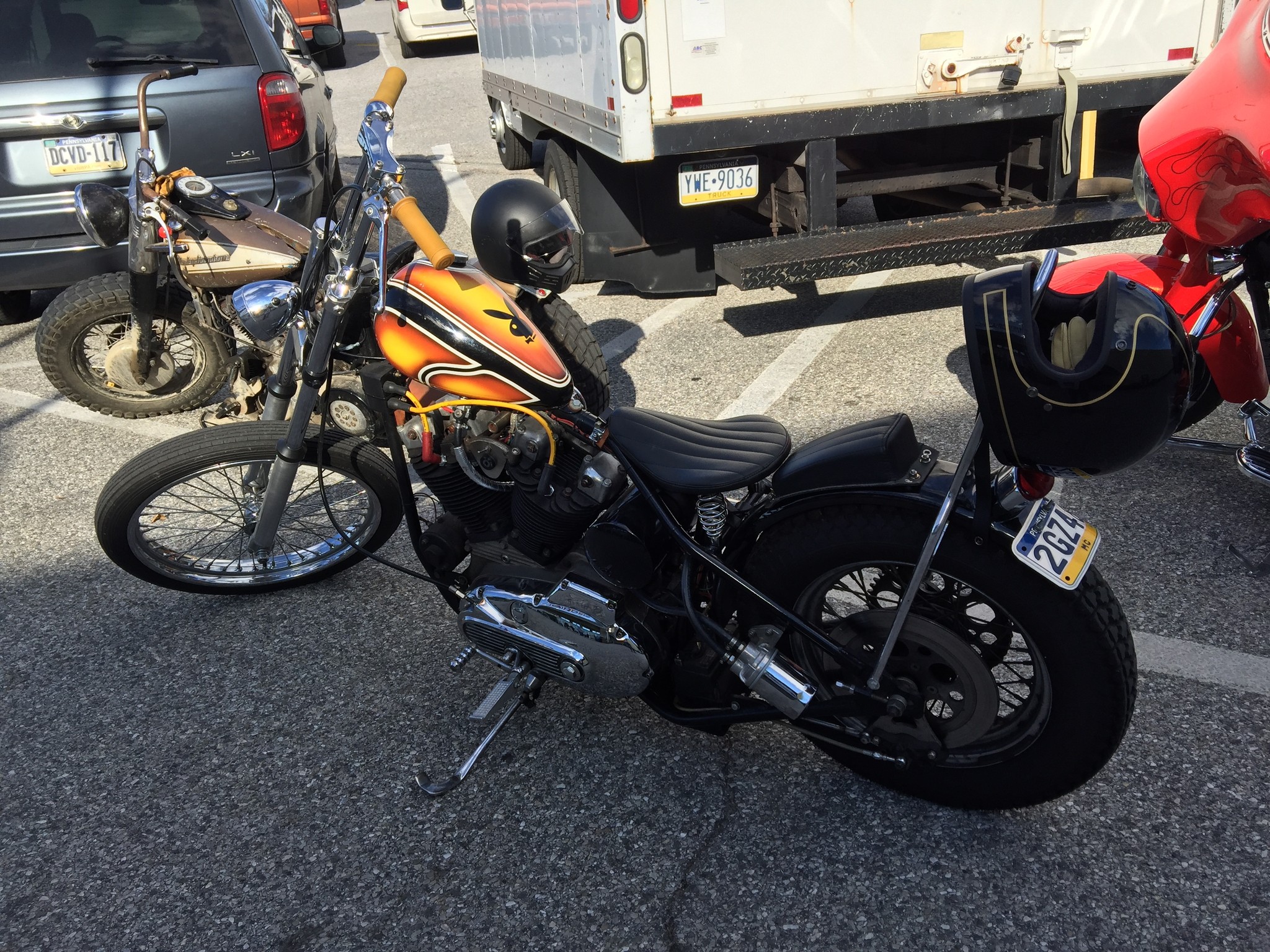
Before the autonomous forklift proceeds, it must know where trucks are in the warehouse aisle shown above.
[474,0,1253,307]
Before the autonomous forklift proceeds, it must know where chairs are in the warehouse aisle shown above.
[48,12,102,77]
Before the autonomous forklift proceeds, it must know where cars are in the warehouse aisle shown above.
[280,0,349,69]
[0,0,347,322]
[389,0,478,58]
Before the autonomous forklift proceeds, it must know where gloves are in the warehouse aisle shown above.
[1048,310,1099,370]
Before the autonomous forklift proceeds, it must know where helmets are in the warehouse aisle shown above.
[469,180,583,292]
[961,265,1195,480]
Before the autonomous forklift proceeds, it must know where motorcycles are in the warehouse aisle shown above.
[91,65,1138,814]
[35,65,470,421]
